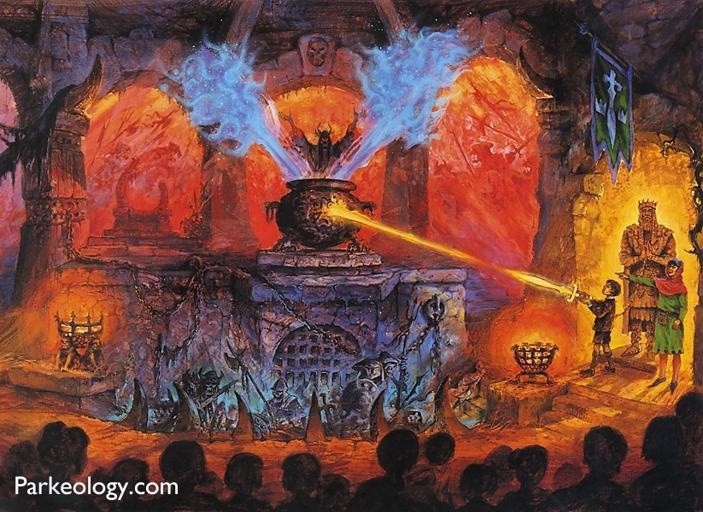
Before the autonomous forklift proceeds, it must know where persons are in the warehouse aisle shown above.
[619,199,676,361]
[577,280,620,377]
[615,260,688,395]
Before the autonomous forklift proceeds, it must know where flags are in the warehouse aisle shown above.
[590,37,634,184]
